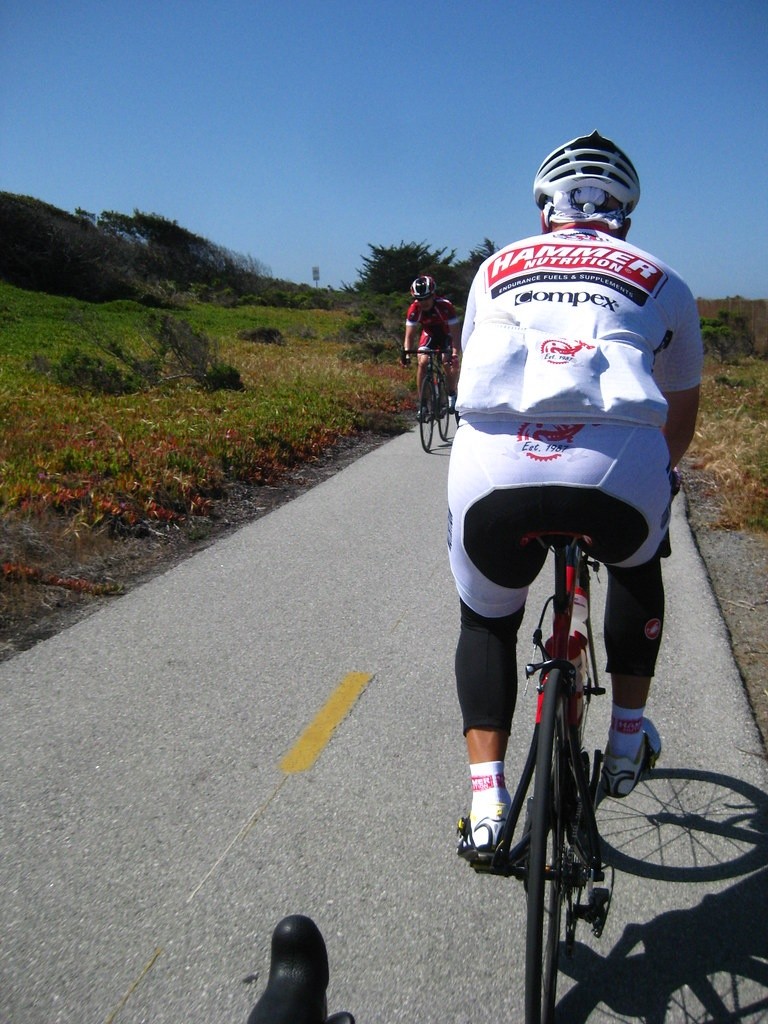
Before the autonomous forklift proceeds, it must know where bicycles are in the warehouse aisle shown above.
[466,521,658,1024]
[400,346,459,453]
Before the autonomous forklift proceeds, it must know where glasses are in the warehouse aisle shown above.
[416,294,431,301]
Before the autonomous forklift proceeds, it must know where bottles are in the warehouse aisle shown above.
[434,379,440,395]
[570,586,589,681]
[534,635,584,720]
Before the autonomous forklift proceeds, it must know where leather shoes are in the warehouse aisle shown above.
[268,915,329,1022]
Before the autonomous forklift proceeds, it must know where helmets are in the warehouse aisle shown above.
[533,129,640,217]
[410,276,435,299]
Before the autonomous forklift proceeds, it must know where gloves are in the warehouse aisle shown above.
[451,354,459,368]
[400,353,411,368]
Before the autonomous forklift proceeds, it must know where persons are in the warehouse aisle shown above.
[447,127,703,864]
[400,275,460,419]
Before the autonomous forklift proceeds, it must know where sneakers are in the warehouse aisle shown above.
[601,717,661,799]
[455,814,507,862]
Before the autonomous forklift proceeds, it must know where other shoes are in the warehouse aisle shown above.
[447,393,456,408]
[418,407,428,419]
